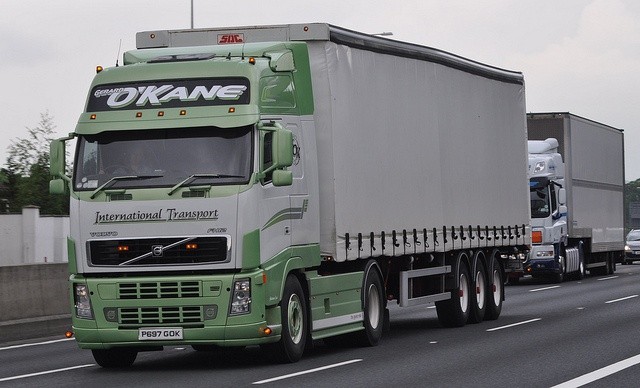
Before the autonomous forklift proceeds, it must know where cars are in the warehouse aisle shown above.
[622,229,640,265]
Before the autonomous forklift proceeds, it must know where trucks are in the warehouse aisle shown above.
[510,112,626,283]
[49,21,532,361]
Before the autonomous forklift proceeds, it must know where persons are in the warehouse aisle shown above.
[112,144,153,176]
